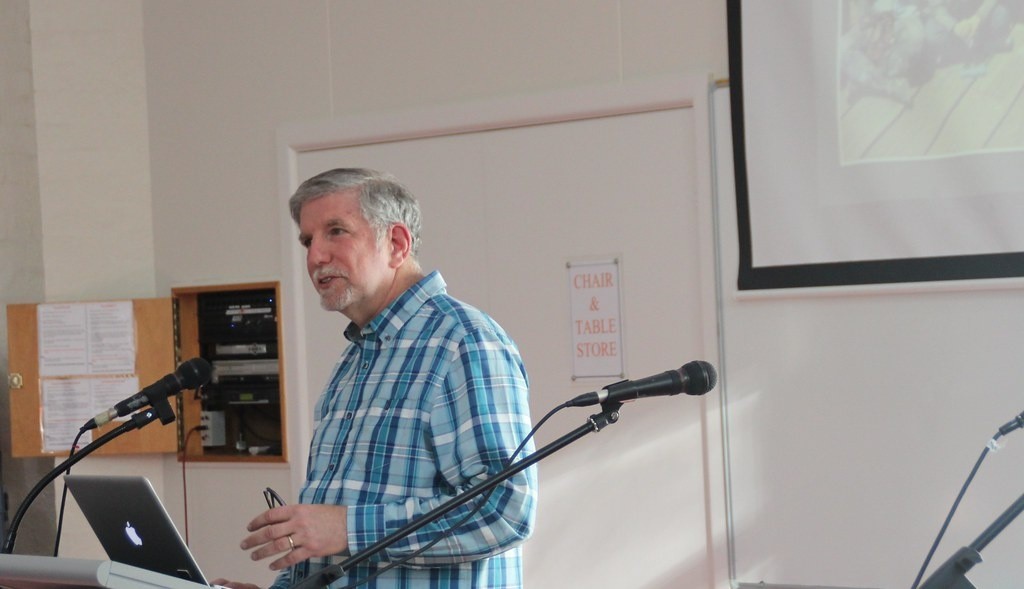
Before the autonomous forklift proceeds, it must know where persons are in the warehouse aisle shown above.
[209,168,537,589]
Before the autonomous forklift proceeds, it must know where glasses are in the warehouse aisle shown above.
[263,486,286,510]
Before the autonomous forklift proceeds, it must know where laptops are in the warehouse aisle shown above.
[62,473,231,589]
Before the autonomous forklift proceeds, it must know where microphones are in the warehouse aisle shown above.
[566,360,717,407]
[84,358,211,430]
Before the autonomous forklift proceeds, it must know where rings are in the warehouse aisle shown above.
[287,535,294,549]
